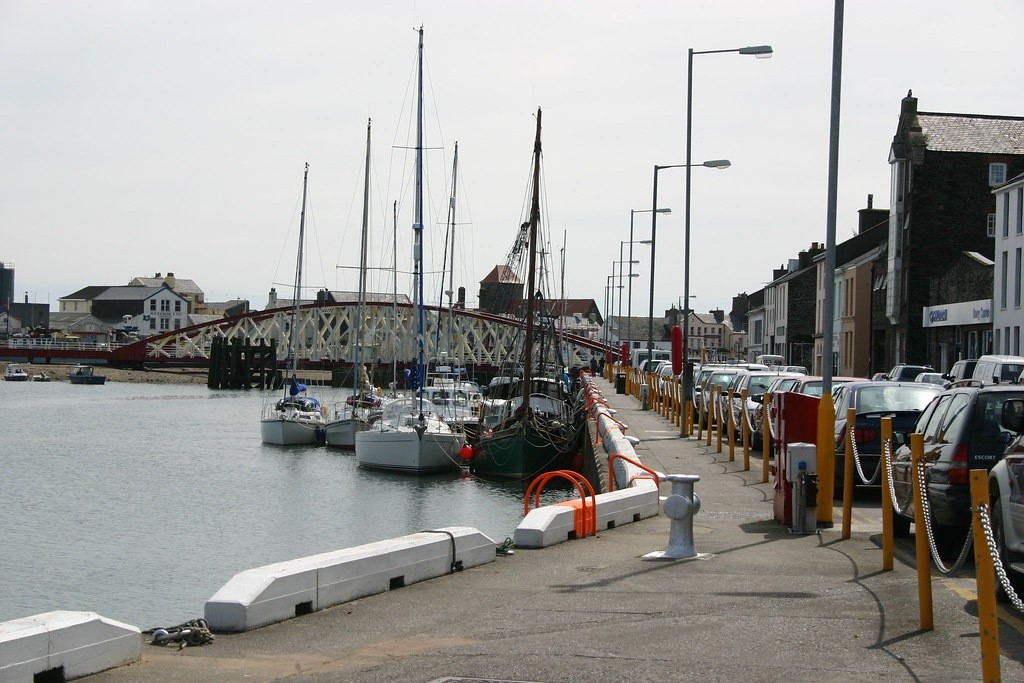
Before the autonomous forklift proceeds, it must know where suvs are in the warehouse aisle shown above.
[893,378,1021,558]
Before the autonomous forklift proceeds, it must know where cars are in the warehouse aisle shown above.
[832,380,947,505]
[624,349,1024,475]
[987,395,1024,603]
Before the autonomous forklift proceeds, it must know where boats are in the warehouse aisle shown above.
[32,371,52,382]
[67,364,107,384]
[3,367,29,381]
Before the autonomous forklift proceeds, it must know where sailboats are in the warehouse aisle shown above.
[259,161,329,448]
[324,19,597,491]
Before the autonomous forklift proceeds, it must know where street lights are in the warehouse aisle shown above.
[679,44,774,440]
[618,240,653,375]
[605,274,639,380]
[604,285,625,380]
[626,208,673,395]
[644,159,733,406]
[609,260,640,384]
[677,295,697,329]
